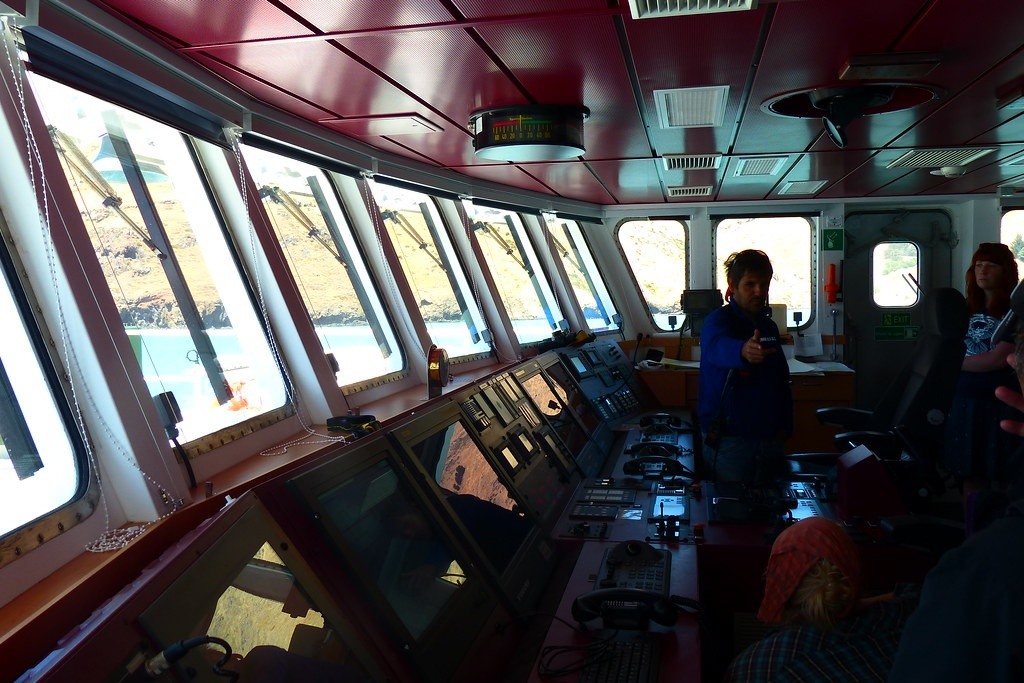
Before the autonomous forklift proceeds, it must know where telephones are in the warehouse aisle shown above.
[623,456,683,476]
[631,441,680,457]
[639,413,681,427]
[570,587,683,632]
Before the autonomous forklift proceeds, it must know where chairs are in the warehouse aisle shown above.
[781,287,967,555]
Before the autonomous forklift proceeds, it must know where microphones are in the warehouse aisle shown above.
[598,332,644,402]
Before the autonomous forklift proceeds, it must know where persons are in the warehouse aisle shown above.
[941,243,1017,513]
[729,517,908,683]
[695,250,795,482]
[885,319,1024,683]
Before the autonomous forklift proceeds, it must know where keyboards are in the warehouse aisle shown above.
[578,628,664,683]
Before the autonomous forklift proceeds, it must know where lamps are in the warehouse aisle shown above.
[808,86,896,150]
